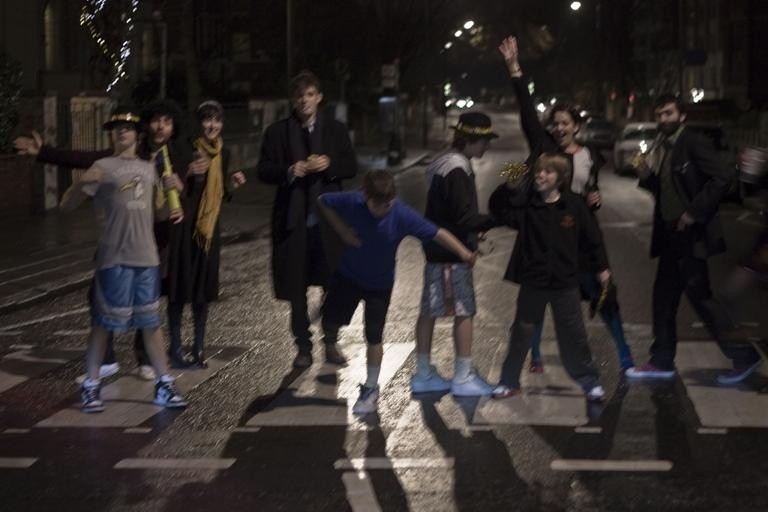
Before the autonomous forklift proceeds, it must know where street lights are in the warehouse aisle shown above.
[153,20,167,101]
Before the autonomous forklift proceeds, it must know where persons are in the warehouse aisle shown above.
[12,101,184,387]
[498,35,636,373]
[256,71,358,369]
[488,152,612,403]
[624,93,765,385]
[315,169,480,413]
[168,99,247,369]
[58,105,190,412]
[409,112,500,396]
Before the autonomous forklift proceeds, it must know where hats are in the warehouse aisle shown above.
[102,106,144,130]
[448,112,499,138]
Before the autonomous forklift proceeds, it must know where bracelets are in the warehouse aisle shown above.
[509,67,521,74]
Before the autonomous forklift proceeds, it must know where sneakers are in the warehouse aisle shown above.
[352,383,379,414]
[293,353,311,368]
[410,364,449,393]
[74,347,207,413]
[624,366,674,378]
[716,350,764,384]
[584,378,605,401]
[450,373,494,396]
[492,382,520,399]
[326,344,346,363]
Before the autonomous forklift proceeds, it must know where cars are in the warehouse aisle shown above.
[649,123,737,200]
[573,113,612,149]
[612,120,658,176]
